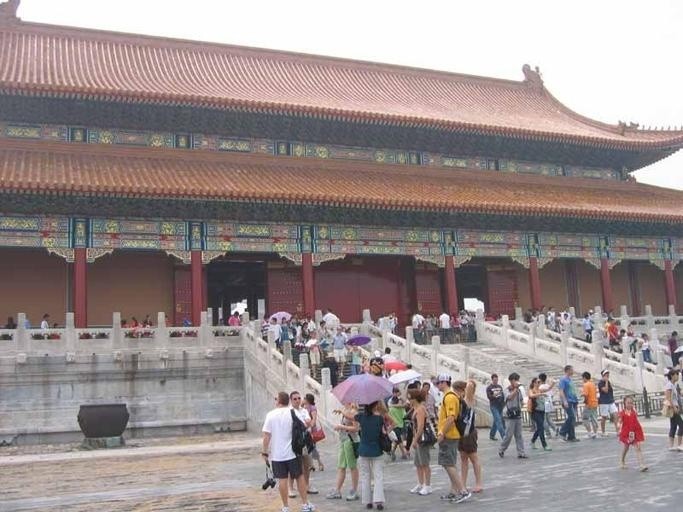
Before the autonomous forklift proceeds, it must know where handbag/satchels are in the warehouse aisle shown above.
[415,426,436,448]
[662,401,674,418]
[354,441,360,457]
[378,418,392,453]
[311,427,325,443]
[506,408,518,418]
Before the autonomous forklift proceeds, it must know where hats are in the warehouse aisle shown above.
[374,350,381,358]
[433,373,452,383]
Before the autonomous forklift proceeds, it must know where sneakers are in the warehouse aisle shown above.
[387,450,411,459]
[307,486,319,494]
[546,427,621,442]
[281,506,290,512]
[289,489,297,498]
[669,447,674,451]
[518,454,528,458]
[621,463,628,469]
[326,492,385,510]
[411,485,484,503]
[530,442,537,449]
[302,500,316,511]
[676,447,682,451]
[497,448,505,458]
[640,465,648,471]
[489,435,498,440]
[543,446,552,451]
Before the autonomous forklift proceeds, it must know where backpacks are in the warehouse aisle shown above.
[443,391,474,436]
[290,409,315,455]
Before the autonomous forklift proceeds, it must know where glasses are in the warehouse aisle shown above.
[293,397,301,400]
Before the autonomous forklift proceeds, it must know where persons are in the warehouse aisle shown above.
[261,308,331,365]
[379,306,500,344]
[664,370,682,451]
[486,366,619,458]
[327,373,483,510]
[130,315,152,338]
[40,313,50,340]
[263,392,325,512]
[665,331,681,370]
[121,319,130,336]
[584,308,657,363]
[165,315,177,337]
[310,326,413,387]
[51,322,59,339]
[183,314,193,336]
[3,316,17,339]
[523,307,572,334]
[614,396,649,471]
[24,315,31,329]
[229,312,239,335]
